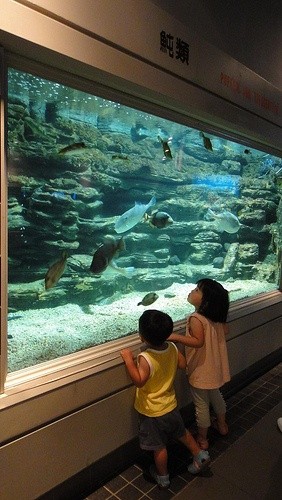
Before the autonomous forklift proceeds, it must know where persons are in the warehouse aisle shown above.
[167,278,231,449]
[120,309,211,487]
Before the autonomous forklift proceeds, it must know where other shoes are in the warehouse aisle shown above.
[217,421,229,435]
[197,436,209,450]
[152,467,170,490]
[186,450,215,477]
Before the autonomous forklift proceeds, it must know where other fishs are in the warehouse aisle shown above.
[45,253,67,290]
[89,195,157,275]
[136,292,160,306]
[51,132,253,234]
